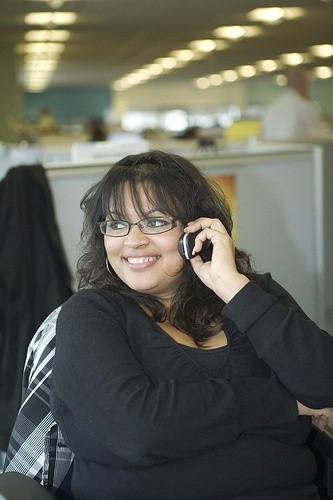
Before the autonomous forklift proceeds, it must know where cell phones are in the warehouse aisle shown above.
[178,229,212,260]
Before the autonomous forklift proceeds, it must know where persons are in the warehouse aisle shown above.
[49,150,333,500]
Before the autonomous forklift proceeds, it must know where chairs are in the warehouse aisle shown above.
[0,413,333,500]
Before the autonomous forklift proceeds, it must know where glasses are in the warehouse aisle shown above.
[97,216,180,238]
